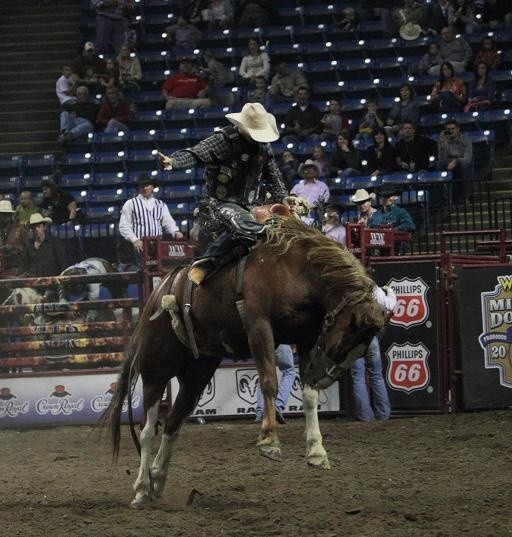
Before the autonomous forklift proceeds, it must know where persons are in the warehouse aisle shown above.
[253,344,296,424]
[351,335,392,422]
[0,0,512,324]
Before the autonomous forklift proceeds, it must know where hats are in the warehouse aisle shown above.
[85,41,94,52]
[398,21,423,41]
[322,194,345,215]
[349,187,376,202]
[130,173,165,187]
[379,183,403,195]
[297,158,322,178]
[29,213,53,226]
[0,200,17,213]
[223,101,281,144]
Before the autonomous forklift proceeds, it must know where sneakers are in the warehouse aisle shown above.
[186,263,209,286]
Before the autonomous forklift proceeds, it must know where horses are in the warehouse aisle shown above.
[0,287,117,371]
[83,214,396,511]
